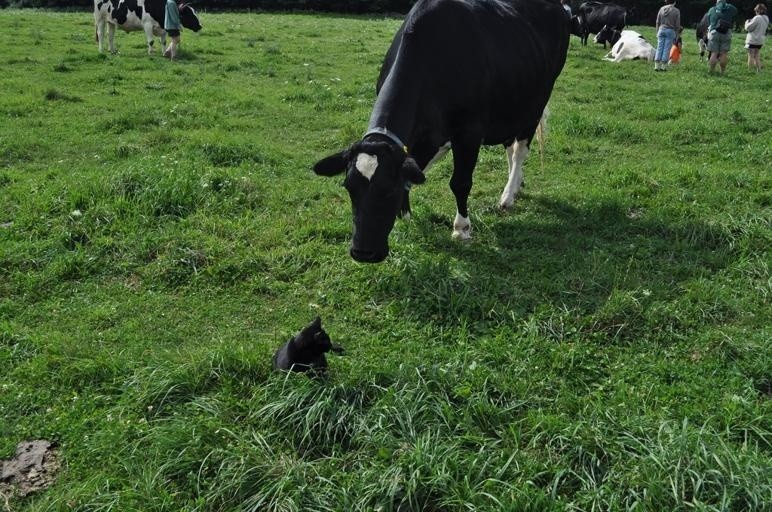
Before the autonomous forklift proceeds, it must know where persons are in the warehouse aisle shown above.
[653,0,682,71]
[694,15,713,65]
[743,3,769,73]
[665,26,684,66]
[706,0,737,76]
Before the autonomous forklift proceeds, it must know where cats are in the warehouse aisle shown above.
[272,316,332,386]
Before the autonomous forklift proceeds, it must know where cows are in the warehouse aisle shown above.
[94,0,202,60]
[313,0,570,263]
[570,1,657,63]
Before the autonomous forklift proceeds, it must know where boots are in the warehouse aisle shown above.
[661,61,668,71]
[654,60,660,71]
